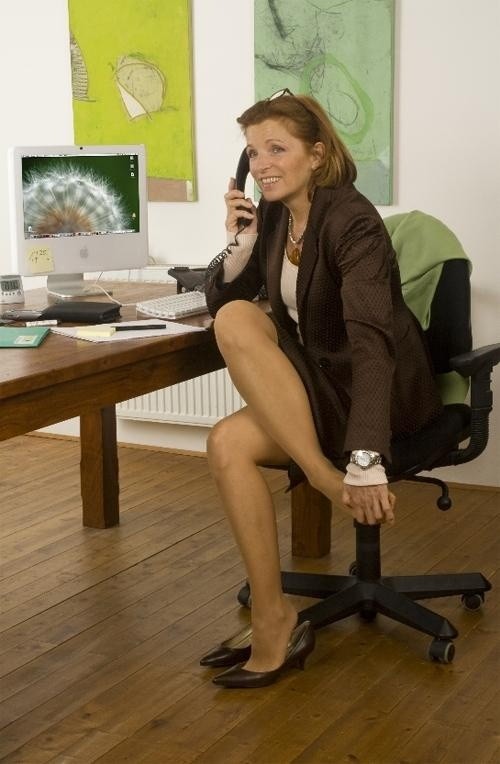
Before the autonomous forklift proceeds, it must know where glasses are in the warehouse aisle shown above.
[263,87,302,106]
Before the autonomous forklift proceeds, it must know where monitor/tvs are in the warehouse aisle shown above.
[9,145,148,298]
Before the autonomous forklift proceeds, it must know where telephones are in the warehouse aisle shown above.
[235,146,252,228]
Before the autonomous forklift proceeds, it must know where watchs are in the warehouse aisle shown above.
[350,450,382,469]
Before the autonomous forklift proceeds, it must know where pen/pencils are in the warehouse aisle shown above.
[114,325,167,331]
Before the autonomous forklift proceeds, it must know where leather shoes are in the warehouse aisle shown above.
[200,620,253,667]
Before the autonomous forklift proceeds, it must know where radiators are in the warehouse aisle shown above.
[81,263,245,428]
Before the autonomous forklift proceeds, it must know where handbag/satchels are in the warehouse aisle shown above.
[43,301,123,324]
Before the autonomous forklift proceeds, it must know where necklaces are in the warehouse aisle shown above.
[287,212,308,266]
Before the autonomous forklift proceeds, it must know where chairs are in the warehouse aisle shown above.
[235,209,500,664]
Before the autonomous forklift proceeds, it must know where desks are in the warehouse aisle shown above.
[0,277,333,560]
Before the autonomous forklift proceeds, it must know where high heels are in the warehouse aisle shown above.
[210,619,316,687]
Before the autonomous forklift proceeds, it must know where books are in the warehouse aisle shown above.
[0,325,50,348]
[50,317,202,345]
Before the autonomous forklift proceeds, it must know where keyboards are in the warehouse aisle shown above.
[136,291,210,320]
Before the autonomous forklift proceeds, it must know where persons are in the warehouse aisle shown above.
[200,89,436,690]
